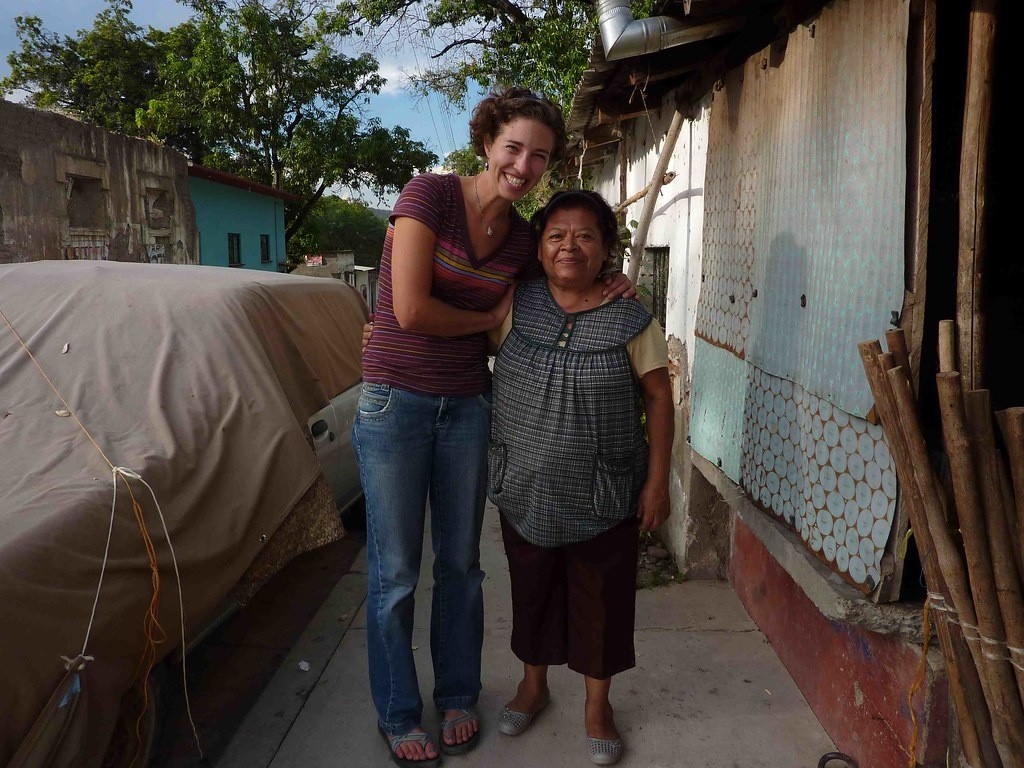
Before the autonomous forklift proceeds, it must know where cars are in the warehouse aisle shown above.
[0,260,366,767]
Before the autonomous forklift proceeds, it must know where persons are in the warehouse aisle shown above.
[353,85,642,768]
[361,191,675,766]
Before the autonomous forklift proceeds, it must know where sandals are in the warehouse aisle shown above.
[376,717,442,767]
[438,707,482,755]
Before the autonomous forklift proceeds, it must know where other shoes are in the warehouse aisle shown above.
[497,705,537,735]
[585,735,623,765]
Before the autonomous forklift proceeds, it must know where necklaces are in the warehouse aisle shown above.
[474,175,509,236]
